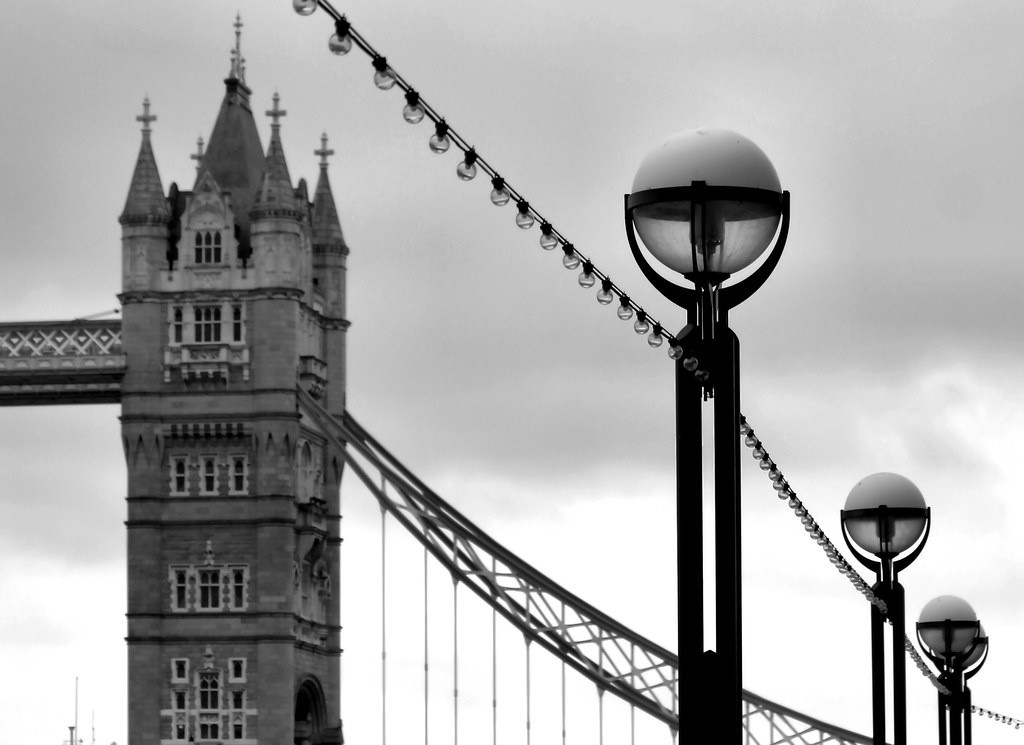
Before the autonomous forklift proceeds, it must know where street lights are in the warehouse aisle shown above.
[623,129,791,745]
[841,472,931,745]
[916,595,989,745]
